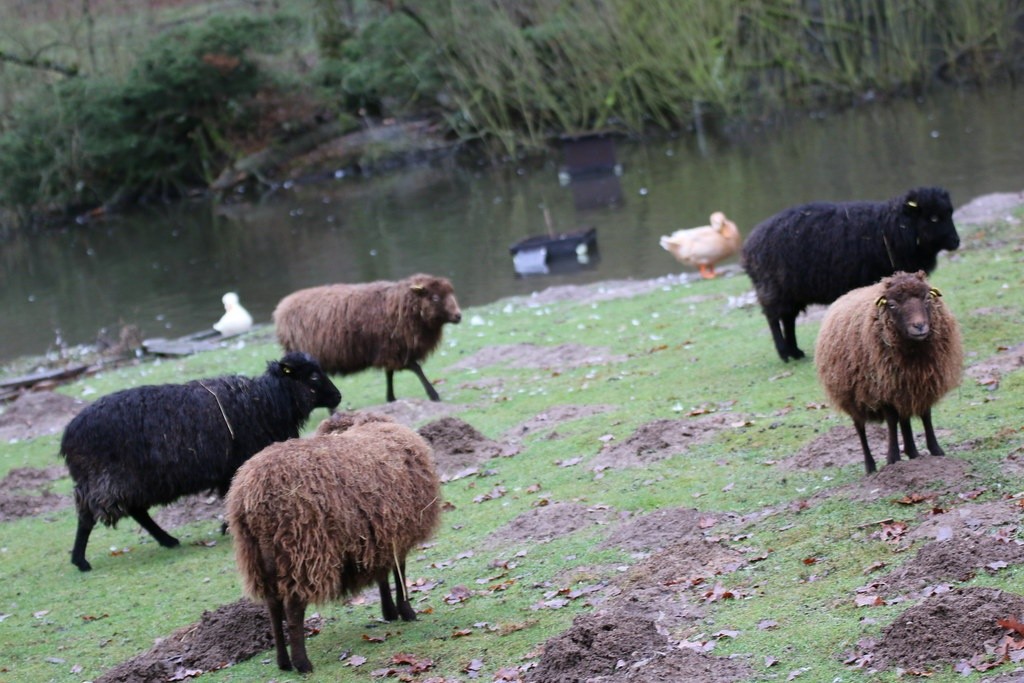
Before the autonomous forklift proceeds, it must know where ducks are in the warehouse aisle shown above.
[212,292,253,340]
[658,210,742,279]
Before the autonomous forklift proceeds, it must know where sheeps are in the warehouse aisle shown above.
[813,270,966,476]
[226,410,441,673]
[58,351,342,574]
[740,185,961,364]
[271,273,462,404]
